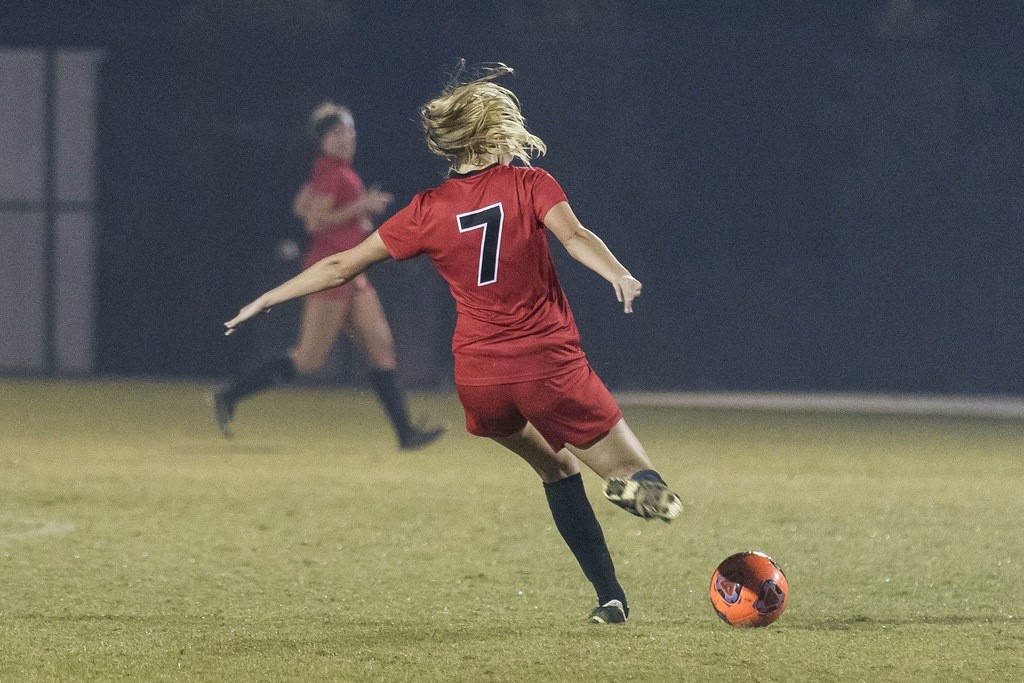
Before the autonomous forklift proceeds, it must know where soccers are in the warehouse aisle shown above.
[709,550,789,629]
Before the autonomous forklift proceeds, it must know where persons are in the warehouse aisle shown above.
[225,83,683,625]
[213,107,450,451]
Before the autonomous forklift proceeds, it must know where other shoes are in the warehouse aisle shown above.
[604,477,685,523]
[399,428,444,449]
[215,387,235,436]
[589,599,629,626]
